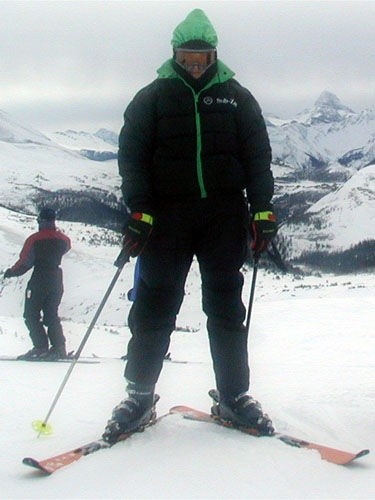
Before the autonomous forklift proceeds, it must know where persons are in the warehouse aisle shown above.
[3,208,71,359]
[100,8,278,440]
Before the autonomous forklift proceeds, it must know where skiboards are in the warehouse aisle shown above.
[0,353,105,364]
[21,404,370,475]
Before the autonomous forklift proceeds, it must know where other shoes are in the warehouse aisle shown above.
[49,344,66,356]
[216,390,264,428]
[26,347,48,358]
[105,382,156,435]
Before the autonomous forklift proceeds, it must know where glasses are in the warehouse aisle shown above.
[173,48,217,73]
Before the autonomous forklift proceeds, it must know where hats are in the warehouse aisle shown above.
[170,8,218,48]
[37,208,56,223]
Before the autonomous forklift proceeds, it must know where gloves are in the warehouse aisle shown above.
[120,209,154,258]
[250,210,278,254]
[4,268,11,279]
